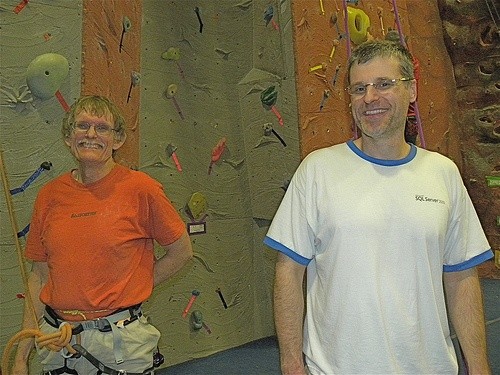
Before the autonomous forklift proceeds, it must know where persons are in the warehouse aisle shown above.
[261,41,494,375]
[384,28,421,147]
[10,94,192,375]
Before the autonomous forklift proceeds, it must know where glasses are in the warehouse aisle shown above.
[69,121,120,135]
[347,77,415,96]
